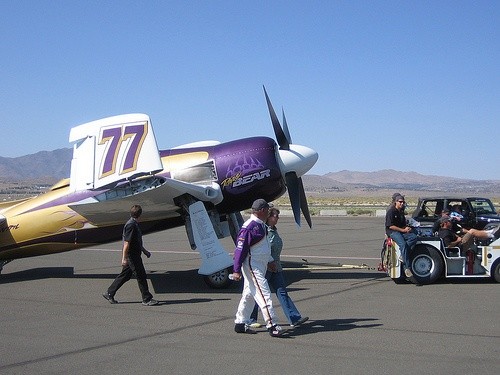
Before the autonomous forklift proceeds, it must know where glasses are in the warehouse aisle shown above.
[398,201,405,204]
[271,214,278,218]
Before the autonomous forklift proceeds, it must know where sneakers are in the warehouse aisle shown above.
[291,317,309,326]
[234,323,256,334]
[103,291,118,304]
[269,325,289,338]
[142,299,159,306]
[248,322,262,328]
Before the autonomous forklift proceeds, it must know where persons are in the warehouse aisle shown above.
[250,208,308,328]
[385,193,500,279]
[102,204,159,306]
[234,198,289,337]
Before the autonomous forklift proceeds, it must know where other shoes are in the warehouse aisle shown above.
[405,269,413,277]
[492,224,500,235]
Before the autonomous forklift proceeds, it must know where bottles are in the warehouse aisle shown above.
[228,274,242,280]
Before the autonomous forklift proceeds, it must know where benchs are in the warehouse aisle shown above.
[440,238,466,260]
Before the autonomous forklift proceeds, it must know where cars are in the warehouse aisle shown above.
[409,195,500,230]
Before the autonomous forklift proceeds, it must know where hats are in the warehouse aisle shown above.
[442,209,450,212]
[441,217,454,223]
[392,193,405,199]
[252,199,274,211]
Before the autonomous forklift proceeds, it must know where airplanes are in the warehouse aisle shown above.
[0,83,320,291]
